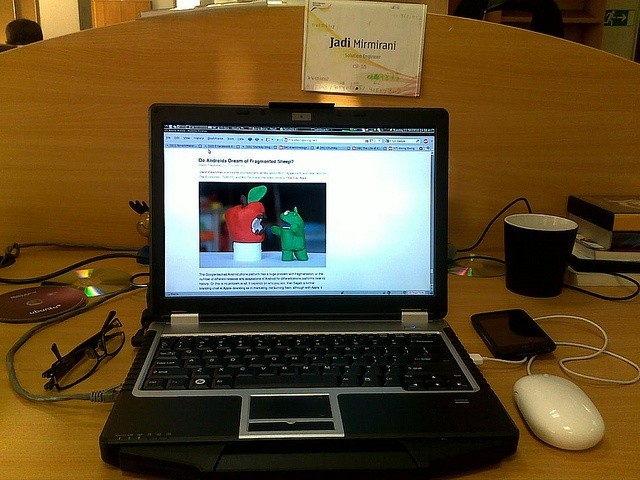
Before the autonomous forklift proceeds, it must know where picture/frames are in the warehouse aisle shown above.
[0,243,639,480]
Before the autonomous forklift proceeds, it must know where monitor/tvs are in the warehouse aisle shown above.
[566,194,640,231]
[563,265,640,287]
[571,233,640,261]
[567,254,640,273]
[565,212,639,252]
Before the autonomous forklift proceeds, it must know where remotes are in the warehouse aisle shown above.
[512,372,605,451]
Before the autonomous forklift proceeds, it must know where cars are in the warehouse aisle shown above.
[504,213,579,298]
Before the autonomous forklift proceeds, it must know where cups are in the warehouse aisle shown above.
[41,310,126,392]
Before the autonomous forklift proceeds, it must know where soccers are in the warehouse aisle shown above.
[223,185,267,243]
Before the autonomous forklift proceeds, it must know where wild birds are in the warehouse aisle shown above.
[99,102,520,476]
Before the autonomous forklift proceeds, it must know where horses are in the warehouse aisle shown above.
[471,308,556,359]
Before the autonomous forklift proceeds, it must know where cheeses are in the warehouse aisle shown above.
[41,267,133,297]
[0,286,86,323]
[448,250,506,278]
